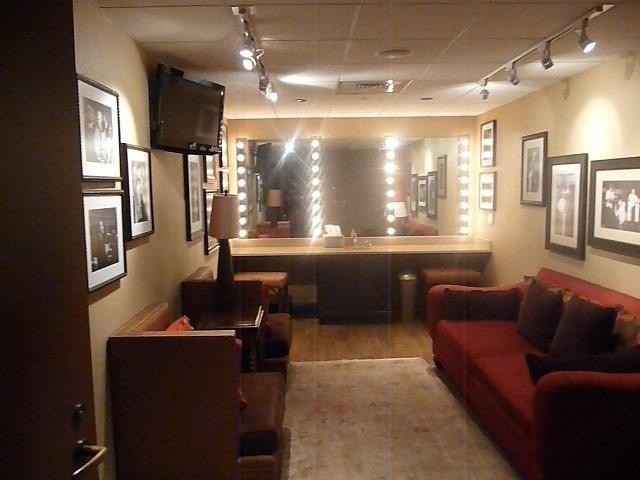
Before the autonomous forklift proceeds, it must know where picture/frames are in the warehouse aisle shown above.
[401,155,450,221]
[80,186,130,295]
[181,117,231,259]
[118,140,156,244]
[544,152,588,261]
[77,71,124,183]
[477,119,498,168]
[519,130,547,209]
[479,171,495,212]
[589,156,639,256]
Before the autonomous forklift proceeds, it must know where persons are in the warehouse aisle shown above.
[605,183,639,224]
[557,192,568,236]
[93,110,108,162]
[93,220,108,270]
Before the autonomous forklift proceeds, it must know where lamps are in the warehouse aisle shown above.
[233,8,278,103]
[476,1,618,101]
[263,183,285,228]
[210,190,243,316]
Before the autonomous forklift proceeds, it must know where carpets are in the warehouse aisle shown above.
[280,355,525,480]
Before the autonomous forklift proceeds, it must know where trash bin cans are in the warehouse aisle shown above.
[397,270,418,323]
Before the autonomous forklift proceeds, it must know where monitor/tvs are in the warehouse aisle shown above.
[150,63,226,153]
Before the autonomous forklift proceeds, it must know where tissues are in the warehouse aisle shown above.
[322,224,344,248]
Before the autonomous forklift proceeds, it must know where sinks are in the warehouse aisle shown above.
[347,246,375,250]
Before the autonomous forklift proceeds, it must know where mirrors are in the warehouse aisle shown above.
[233,136,472,237]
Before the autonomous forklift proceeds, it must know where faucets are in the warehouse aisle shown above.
[351,229,355,236]
[351,233,358,245]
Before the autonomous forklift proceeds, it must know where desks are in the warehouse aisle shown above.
[187,305,267,373]
[228,239,495,325]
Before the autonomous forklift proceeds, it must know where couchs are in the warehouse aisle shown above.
[180,266,295,358]
[425,266,640,479]
[105,299,288,480]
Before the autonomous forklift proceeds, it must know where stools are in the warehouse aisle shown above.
[235,271,295,319]
[421,266,485,290]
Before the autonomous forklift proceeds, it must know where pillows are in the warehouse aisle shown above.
[517,280,640,389]
[167,314,196,333]
